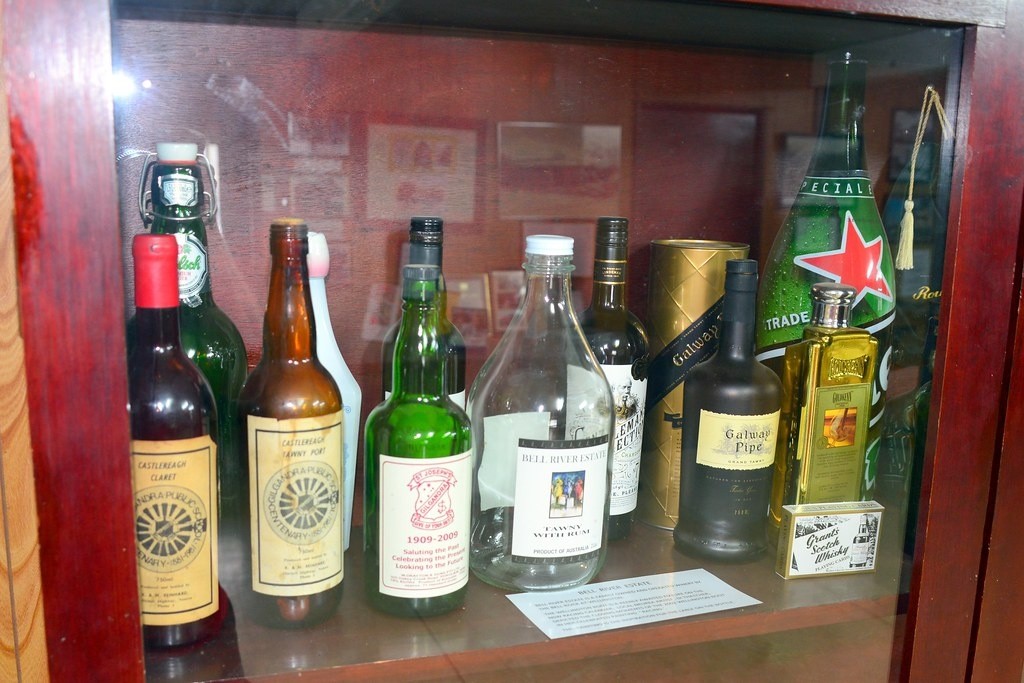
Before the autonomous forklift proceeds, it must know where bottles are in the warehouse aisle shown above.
[576,218,649,546]
[766,284,879,548]
[465,234,614,594]
[671,258,783,564]
[364,263,474,617]
[754,51,897,502]
[306,231,363,551]
[234,218,345,631]
[381,216,467,410]
[127,143,251,595]
[125,232,224,657]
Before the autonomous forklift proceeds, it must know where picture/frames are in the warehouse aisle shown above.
[887,106,934,182]
[495,121,621,219]
[351,111,484,234]
[777,130,863,210]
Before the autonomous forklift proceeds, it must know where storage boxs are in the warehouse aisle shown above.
[774,500,885,580]
